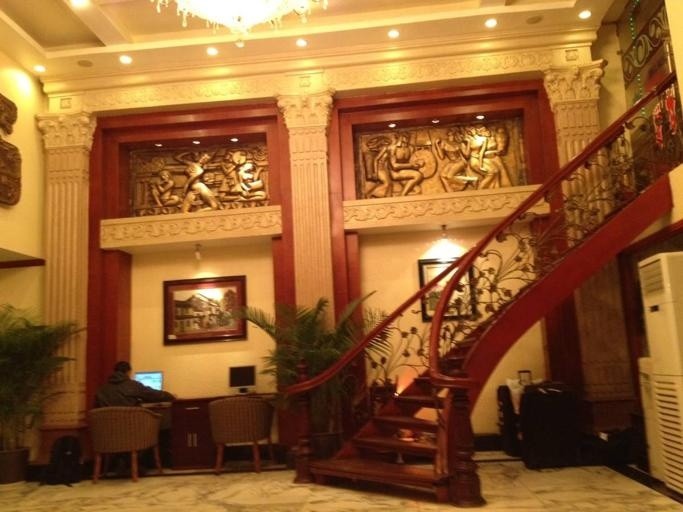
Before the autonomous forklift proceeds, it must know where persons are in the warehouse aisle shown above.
[219,152,243,205]
[389,132,425,194]
[234,162,268,203]
[458,125,500,189]
[362,136,389,200]
[174,147,219,214]
[151,170,181,214]
[475,123,513,187]
[91,359,177,408]
[435,127,467,193]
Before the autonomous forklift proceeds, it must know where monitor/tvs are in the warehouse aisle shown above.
[229,365,256,395]
[135,371,164,391]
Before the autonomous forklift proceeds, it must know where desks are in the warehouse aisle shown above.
[139,392,280,468]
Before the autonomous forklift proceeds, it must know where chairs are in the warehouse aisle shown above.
[208,396,273,475]
[89,405,164,483]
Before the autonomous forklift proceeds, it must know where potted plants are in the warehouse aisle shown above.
[0,302,90,484]
[217,288,394,469]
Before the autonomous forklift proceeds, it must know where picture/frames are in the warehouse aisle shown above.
[416,257,480,324]
[161,275,247,345]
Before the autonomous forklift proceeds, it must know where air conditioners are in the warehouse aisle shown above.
[637,250,682,495]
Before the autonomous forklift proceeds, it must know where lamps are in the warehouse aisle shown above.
[194,243,202,261]
[145,0,333,34]
